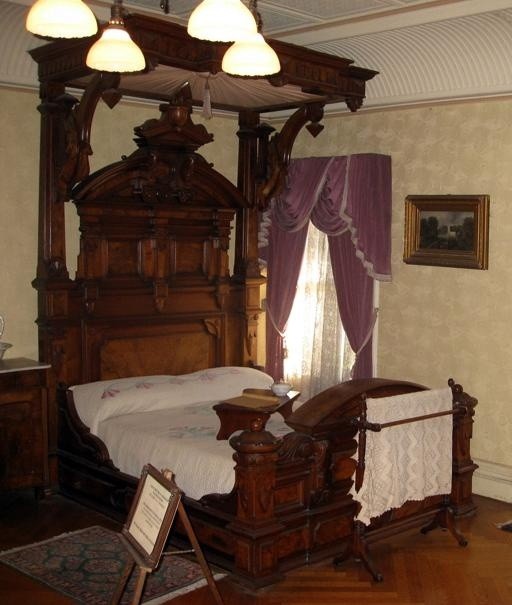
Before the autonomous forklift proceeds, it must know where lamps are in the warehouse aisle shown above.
[186,0,258,42]
[86,0,146,73]
[222,5,279,77]
[25,1,98,39]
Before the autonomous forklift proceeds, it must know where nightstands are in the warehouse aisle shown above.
[0,358,53,497]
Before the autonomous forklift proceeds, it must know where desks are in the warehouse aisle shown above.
[213,389,301,441]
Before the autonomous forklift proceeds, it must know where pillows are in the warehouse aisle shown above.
[69,365,273,435]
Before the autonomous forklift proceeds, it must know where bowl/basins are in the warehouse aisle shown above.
[272,384,291,396]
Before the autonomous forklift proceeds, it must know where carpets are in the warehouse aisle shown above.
[0,525,228,605]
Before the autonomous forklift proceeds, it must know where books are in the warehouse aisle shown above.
[223,388,280,411]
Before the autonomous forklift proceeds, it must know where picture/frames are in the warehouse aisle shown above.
[402,194,493,271]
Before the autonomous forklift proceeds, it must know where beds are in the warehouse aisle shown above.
[27,13,478,588]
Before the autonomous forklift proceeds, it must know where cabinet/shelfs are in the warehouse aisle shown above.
[336,378,468,582]
